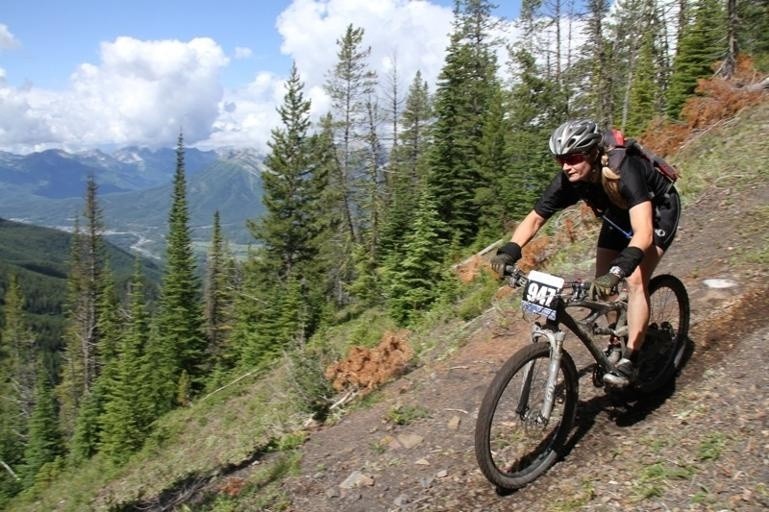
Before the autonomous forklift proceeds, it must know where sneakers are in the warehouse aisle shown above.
[603,357,640,389]
[600,338,622,363]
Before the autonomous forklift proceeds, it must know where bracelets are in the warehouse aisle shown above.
[607,245,644,277]
[496,242,522,260]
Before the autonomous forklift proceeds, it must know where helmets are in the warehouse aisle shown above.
[549,118,602,155]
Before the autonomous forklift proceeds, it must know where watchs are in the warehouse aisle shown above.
[608,266,623,280]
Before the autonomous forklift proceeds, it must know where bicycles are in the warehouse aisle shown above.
[474,257,691,492]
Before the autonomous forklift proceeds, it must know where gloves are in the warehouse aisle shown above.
[489,242,522,280]
[590,271,620,301]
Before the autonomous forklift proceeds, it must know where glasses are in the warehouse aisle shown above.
[556,154,585,166]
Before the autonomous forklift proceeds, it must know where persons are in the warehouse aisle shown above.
[488,116,682,387]
